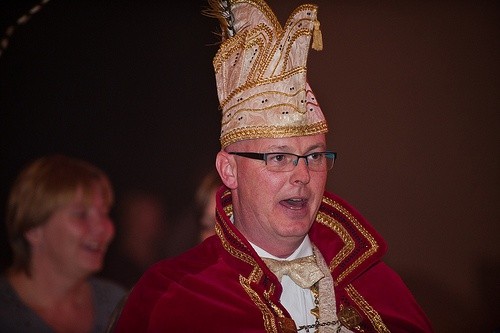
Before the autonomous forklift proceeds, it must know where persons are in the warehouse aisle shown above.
[110,0,434,333]
[0,156,131,333]
[111,189,195,286]
[195,168,222,241]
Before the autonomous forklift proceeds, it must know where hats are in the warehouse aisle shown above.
[201,0,329,150]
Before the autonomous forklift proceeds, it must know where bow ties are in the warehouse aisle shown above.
[262,257,324,290]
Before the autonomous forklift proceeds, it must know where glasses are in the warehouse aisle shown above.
[228,147,337,172]
